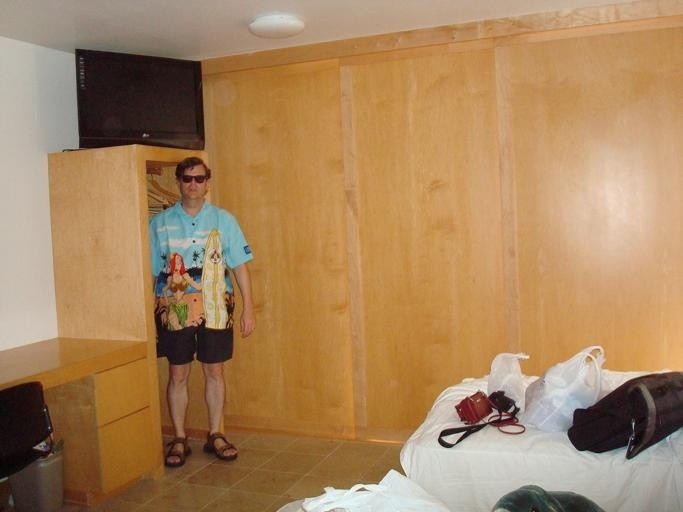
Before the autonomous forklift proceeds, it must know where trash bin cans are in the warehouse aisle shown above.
[9,451,64,512]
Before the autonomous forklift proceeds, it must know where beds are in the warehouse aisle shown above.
[400,373,681,510]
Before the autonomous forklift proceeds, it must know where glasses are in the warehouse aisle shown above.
[182,175,207,183]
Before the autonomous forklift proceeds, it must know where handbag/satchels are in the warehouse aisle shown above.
[569,372,683,459]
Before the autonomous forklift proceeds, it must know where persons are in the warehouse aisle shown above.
[149,157,256,468]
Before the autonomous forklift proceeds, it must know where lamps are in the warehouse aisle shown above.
[248,14,306,39]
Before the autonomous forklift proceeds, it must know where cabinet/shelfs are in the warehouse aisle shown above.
[0,336,152,512]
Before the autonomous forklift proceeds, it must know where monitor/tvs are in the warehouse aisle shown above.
[75,48,205,152]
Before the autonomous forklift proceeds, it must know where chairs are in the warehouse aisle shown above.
[0,382,56,482]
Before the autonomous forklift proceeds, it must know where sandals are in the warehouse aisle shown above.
[166,434,192,467]
[204,431,238,460]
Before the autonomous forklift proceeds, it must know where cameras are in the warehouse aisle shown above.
[488,392,516,413]
